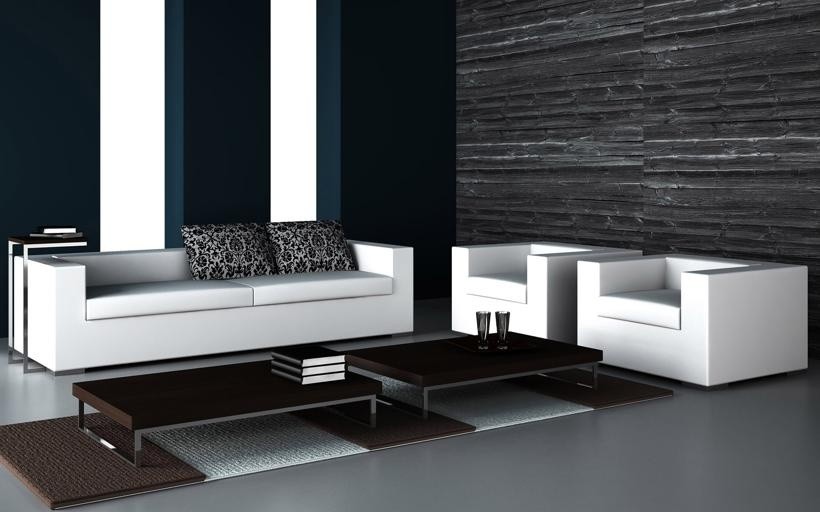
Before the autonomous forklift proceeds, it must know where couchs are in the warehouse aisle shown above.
[9,236,416,375]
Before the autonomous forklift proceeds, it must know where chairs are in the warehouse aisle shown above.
[448,241,812,388]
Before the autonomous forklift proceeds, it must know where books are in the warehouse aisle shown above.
[27,225,83,239]
[271,347,347,385]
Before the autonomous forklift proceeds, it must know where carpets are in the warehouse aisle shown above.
[2,364,675,507]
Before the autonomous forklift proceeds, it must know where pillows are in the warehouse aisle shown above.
[181,219,358,280]
